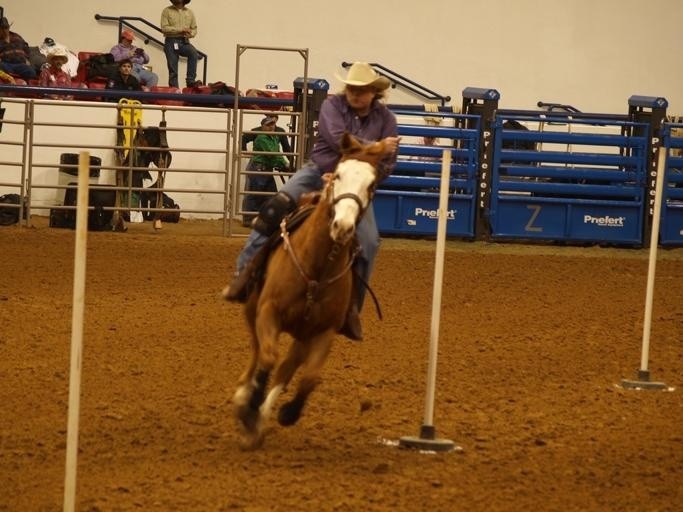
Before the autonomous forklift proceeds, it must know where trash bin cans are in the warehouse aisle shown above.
[56,153,101,206]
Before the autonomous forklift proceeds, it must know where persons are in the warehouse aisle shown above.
[240,114,295,228]
[407,135,453,192]
[161,0,204,89]
[220,61,400,336]
[0,17,36,82]
[110,28,159,92]
[101,58,148,104]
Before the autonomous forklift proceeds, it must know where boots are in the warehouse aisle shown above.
[222,274,238,302]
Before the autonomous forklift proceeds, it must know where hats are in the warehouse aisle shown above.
[0,16,14,31]
[46,46,68,63]
[170,2,190,6]
[261,118,275,127]
[45,36,55,46]
[335,60,390,90]
[122,28,136,41]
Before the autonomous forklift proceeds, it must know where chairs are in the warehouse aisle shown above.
[0,51,295,113]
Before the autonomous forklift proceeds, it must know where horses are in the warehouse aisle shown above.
[228,129,403,451]
